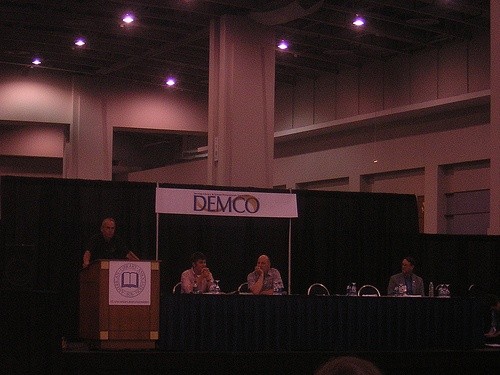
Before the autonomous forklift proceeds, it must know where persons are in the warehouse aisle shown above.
[82,218,141,268]
[247,255,284,294]
[484,295,500,337]
[388,257,424,297]
[181,252,217,293]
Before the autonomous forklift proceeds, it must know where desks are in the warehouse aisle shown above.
[159,293,467,350]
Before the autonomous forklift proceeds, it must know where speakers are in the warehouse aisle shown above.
[0,245,35,302]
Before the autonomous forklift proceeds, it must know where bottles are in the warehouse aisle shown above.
[273,279,280,295]
[412,281,417,294]
[192,281,199,294]
[429,282,434,297]
